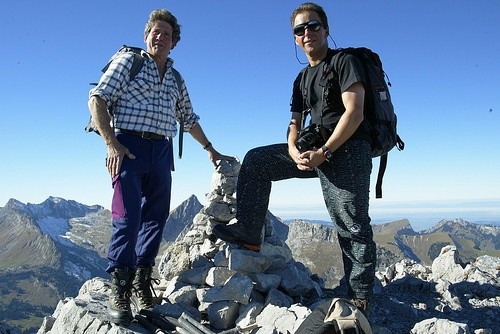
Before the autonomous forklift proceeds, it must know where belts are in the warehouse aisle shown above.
[113,128,172,142]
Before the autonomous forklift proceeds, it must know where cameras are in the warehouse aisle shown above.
[295,123,327,153]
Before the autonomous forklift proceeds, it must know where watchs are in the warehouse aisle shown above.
[322,146,331,158]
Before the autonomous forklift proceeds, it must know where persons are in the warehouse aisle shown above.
[88,9,236,323]
[212,2,376,318]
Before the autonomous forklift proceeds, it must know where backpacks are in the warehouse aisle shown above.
[300,47,405,198]
[84,44,182,136]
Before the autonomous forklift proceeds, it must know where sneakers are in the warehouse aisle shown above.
[351,298,369,318]
[212,222,263,252]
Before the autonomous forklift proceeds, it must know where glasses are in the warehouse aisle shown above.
[294,19,328,38]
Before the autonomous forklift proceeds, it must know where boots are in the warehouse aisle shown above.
[130,268,153,312]
[107,268,136,326]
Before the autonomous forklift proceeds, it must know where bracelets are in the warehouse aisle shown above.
[203,142,211,149]
